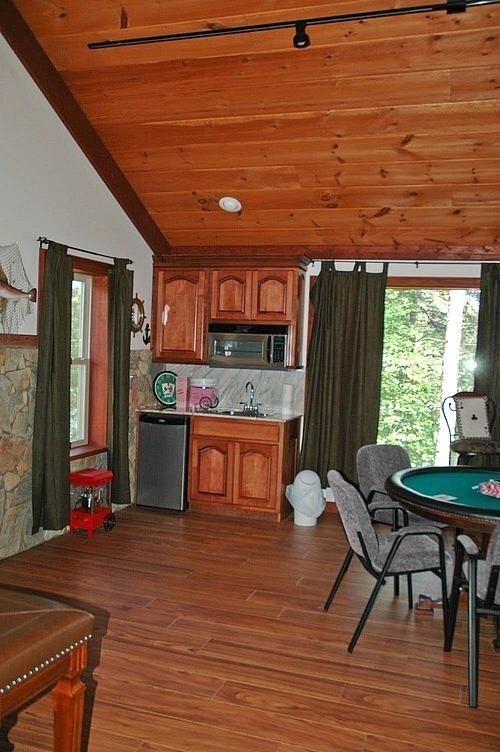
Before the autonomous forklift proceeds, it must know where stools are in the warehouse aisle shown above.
[2,585,94,752]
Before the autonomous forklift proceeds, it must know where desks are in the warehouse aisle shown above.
[384,463,500,652]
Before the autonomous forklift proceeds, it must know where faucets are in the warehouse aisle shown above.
[244,381,254,412]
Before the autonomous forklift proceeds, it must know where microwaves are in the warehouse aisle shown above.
[207,332,289,371]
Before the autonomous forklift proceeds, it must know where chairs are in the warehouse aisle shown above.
[443,529,500,708]
[322,469,457,653]
[441,392,500,466]
[356,444,450,598]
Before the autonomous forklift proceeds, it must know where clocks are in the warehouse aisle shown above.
[153,370,179,409]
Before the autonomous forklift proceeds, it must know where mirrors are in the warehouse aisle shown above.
[130,292,145,338]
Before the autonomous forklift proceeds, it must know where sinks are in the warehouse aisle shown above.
[216,411,273,418]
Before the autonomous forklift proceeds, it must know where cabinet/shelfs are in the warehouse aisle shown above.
[209,267,297,324]
[150,264,210,366]
[188,413,302,522]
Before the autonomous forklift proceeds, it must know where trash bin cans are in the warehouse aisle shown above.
[292,469,318,526]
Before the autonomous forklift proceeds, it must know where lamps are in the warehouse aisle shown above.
[290,18,312,50]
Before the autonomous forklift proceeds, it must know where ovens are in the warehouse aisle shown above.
[136,413,189,513]
